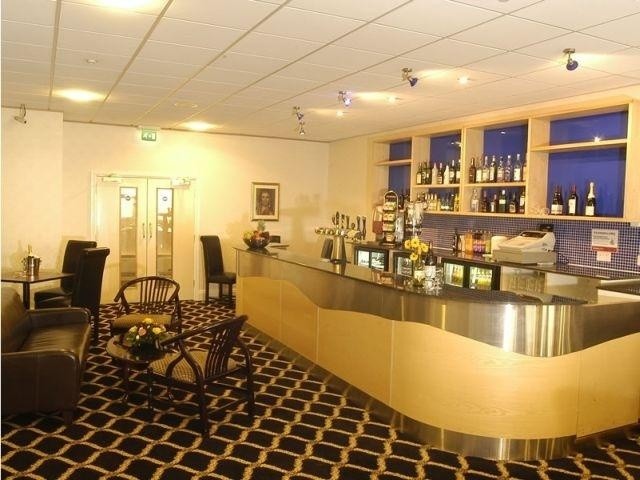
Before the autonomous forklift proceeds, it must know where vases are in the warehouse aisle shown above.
[136,340,157,362]
[411,262,428,287]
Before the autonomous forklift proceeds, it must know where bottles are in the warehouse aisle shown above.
[584,182,596,217]
[412,250,425,287]
[450,266,492,290]
[416,159,460,184]
[469,153,526,182]
[417,192,459,212]
[470,187,525,213]
[566,185,578,216]
[452,229,491,253]
[550,183,564,215]
[27,244,35,258]
[426,241,436,280]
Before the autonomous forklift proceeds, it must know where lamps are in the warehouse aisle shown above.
[338,90,353,106]
[398,68,423,90]
[563,47,582,72]
[290,105,307,136]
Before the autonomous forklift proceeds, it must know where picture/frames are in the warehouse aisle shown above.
[249,181,281,221]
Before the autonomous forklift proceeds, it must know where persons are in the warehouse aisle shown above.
[256,192,275,215]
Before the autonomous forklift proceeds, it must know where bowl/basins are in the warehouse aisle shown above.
[244,239,270,249]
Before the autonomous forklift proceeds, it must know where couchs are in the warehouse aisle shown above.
[2,286,92,425]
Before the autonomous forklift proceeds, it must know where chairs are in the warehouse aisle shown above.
[36,246,111,341]
[148,313,255,437]
[109,274,183,340]
[199,233,237,307]
[33,238,98,310]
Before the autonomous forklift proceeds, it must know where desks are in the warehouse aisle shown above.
[1,270,76,310]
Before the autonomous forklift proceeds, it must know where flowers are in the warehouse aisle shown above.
[129,317,168,339]
[403,239,428,262]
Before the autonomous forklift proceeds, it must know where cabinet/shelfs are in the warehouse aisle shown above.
[353,245,388,273]
[439,257,500,292]
[371,95,639,225]
[393,252,438,281]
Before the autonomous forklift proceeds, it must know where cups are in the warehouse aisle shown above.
[422,270,435,293]
[508,269,544,294]
[400,258,412,277]
[432,267,445,289]
